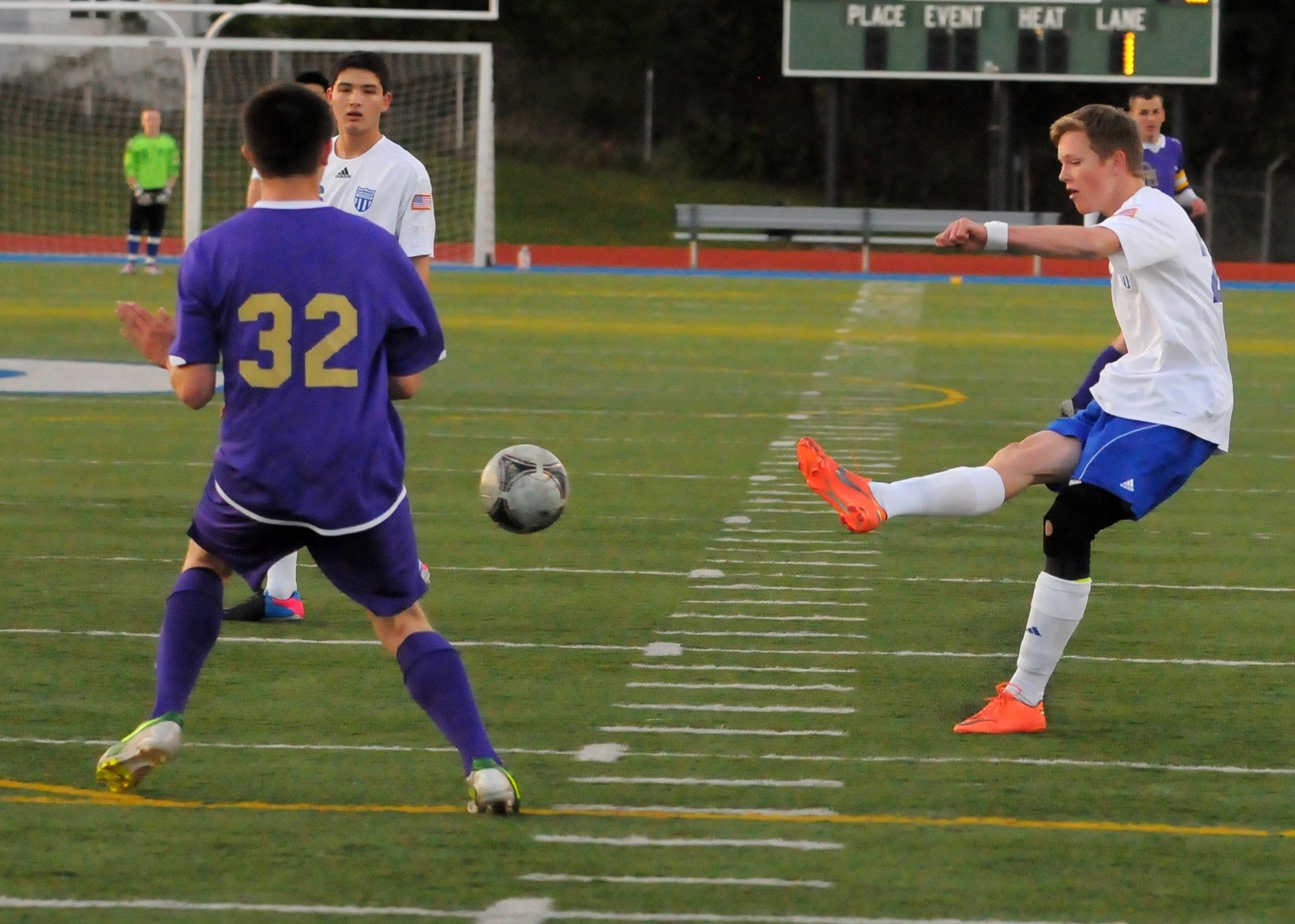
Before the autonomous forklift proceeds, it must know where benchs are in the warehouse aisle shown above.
[670,204,1061,277]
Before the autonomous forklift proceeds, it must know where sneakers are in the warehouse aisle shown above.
[220,588,304,623]
[466,757,521,814]
[952,681,1046,734]
[795,436,886,535]
[94,711,184,794]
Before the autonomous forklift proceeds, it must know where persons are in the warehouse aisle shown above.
[797,105,1233,734]
[246,71,331,208]
[96,88,519,814]
[120,109,180,273]
[225,51,436,622]
[1060,89,1208,416]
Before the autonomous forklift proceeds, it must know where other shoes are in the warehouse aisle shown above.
[120,263,136,275]
[145,265,161,275]
[1059,396,1077,419]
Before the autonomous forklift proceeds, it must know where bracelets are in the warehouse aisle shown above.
[984,221,1008,250]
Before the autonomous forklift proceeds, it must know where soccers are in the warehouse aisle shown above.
[479,443,569,534]
